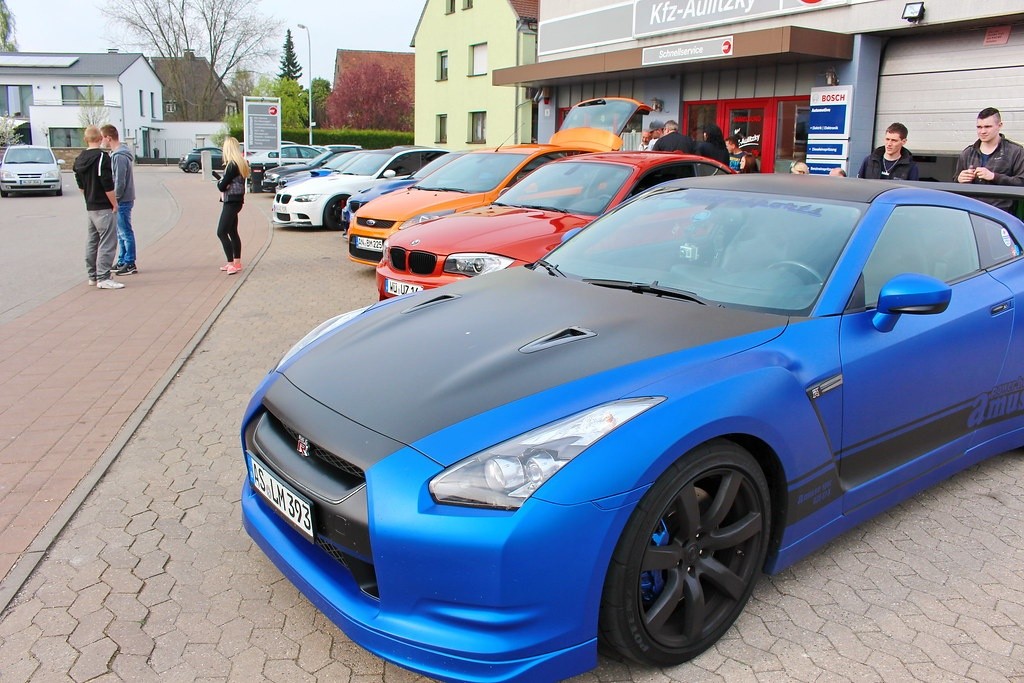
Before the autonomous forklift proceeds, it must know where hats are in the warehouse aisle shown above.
[648,120,664,134]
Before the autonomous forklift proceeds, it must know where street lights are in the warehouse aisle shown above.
[297,24,312,146]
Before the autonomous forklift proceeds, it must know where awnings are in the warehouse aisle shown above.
[143,126,164,130]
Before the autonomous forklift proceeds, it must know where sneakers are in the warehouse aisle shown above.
[220,264,241,271]
[110,263,124,273]
[97,279,125,289]
[89,279,97,286]
[116,264,138,276]
[227,262,237,275]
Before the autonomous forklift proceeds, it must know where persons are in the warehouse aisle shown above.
[216,136,249,274]
[952,108,1024,213]
[790,160,809,174]
[72,124,126,289]
[101,124,138,276]
[638,120,760,174]
[830,168,846,177]
[858,122,919,180]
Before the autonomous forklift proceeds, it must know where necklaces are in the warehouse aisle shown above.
[882,153,901,176]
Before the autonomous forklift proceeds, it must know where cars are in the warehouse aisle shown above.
[272,144,452,231]
[341,150,471,240]
[0,145,66,197]
[374,151,740,305]
[347,96,657,266]
[178,147,226,174]
[239,141,369,195]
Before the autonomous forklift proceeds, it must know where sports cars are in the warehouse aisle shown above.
[240,175,1024,683]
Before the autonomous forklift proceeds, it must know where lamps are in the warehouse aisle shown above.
[528,22,538,32]
[824,67,839,86]
[902,2,926,23]
[650,97,663,111]
